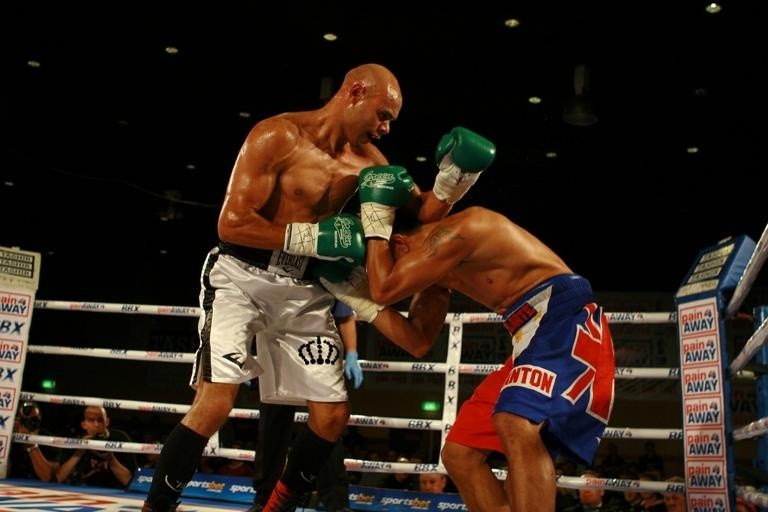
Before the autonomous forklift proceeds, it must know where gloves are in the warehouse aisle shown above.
[285,212,367,267]
[432,127,496,204]
[359,165,413,241]
[342,349,365,390]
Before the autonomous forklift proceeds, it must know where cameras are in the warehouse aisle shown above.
[20,402,41,431]
[92,432,107,454]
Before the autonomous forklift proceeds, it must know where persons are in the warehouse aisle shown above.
[5,396,142,489]
[554,468,768,510]
[249,298,366,512]
[345,450,446,494]
[136,60,499,512]
[316,161,617,511]
